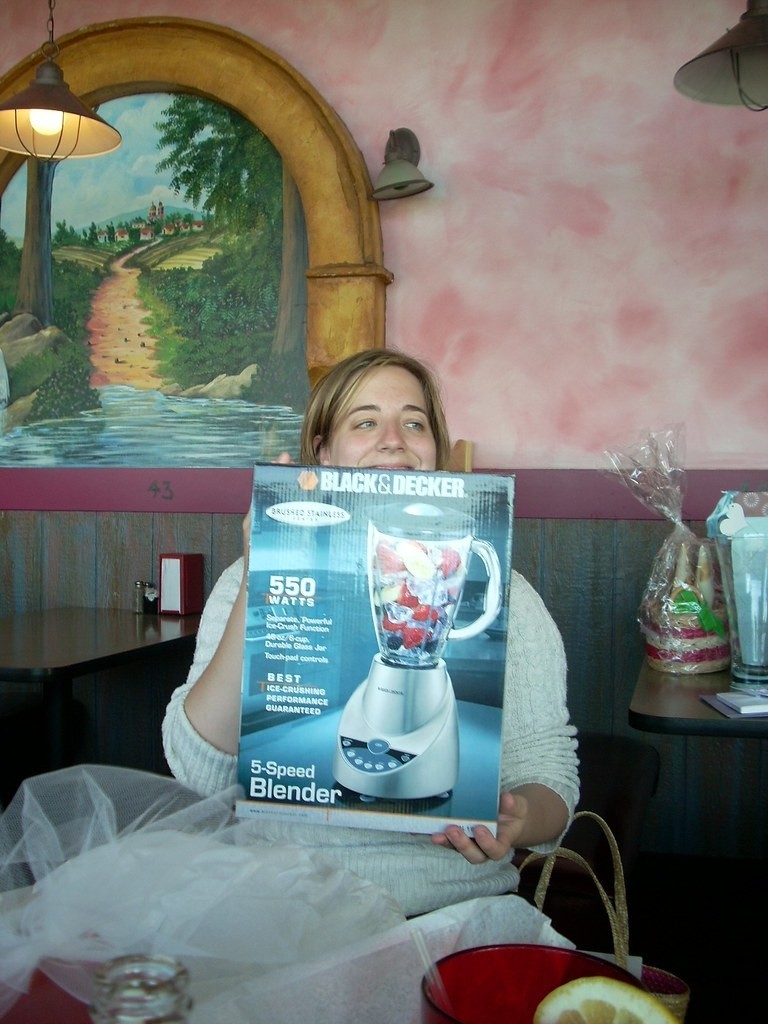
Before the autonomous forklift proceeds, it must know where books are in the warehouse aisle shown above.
[716,691,768,714]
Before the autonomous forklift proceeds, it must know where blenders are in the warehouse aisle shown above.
[330,503,504,800]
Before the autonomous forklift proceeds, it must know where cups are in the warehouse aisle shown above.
[419,943,653,1024]
[717,533,768,699]
[641,965,690,1024]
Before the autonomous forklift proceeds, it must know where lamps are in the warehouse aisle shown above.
[367,127,434,200]
[0,0,122,164]
[673,0,768,112]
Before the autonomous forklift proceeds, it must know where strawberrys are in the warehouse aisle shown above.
[370,540,461,648]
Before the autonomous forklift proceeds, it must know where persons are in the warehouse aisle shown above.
[162,347,579,923]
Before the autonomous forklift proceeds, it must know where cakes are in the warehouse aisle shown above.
[640,539,734,675]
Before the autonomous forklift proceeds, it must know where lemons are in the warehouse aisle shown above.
[531,976,679,1024]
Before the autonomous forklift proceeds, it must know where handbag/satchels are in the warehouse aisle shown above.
[516,810,690,1023]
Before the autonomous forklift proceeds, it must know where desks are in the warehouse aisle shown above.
[627,655,768,739]
[0,605,202,768]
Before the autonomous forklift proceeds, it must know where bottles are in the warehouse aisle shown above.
[132,581,158,614]
[87,953,195,1024]
